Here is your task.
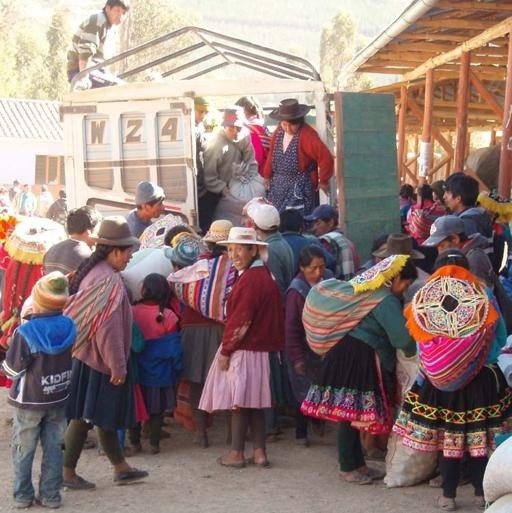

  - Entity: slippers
[245,455,270,468]
[216,454,246,468]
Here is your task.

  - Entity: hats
[165,198,340,264]
[88,216,142,250]
[32,269,71,313]
[370,234,424,263]
[425,214,467,246]
[221,106,248,128]
[267,99,311,123]
[134,180,165,205]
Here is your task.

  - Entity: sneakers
[34,492,62,509]
[113,467,149,485]
[62,475,97,491]
[59,418,324,453]
[14,493,34,508]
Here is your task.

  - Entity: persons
[67,0,129,90]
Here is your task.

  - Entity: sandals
[429,464,484,512]
[360,444,386,461]
[373,439,387,450]
[340,471,372,485]
[357,464,387,479]
